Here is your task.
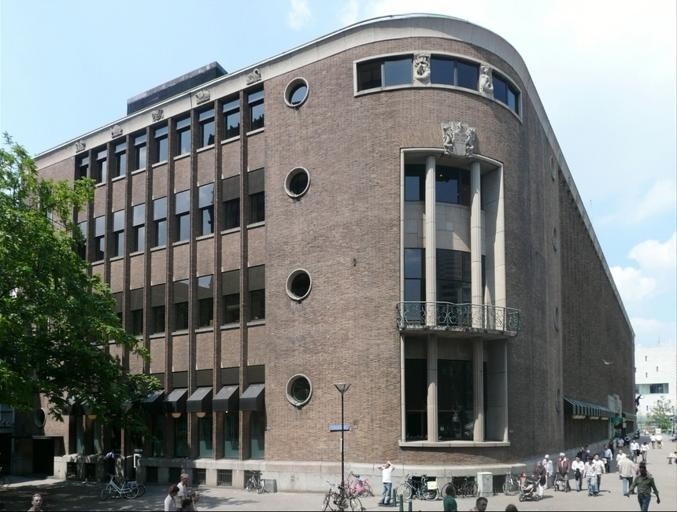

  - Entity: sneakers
[576,489,599,496]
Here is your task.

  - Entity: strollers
[552,470,572,493]
[516,474,545,502]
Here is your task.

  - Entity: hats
[545,454,549,459]
[560,452,565,457]
[181,474,189,479]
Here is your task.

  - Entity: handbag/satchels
[182,495,193,509]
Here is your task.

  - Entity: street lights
[331,382,352,498]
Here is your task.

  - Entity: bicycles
[440,475,479,500]
[395,473,440,503]
[498,470,532,497]
[321,470,371,512]
[97,471,146,500]
[244,468,266,494]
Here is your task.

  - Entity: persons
[175,473,200,512]
[27,493,44,511]
[443,486,457,511]
[469,497,488,512]
[537,429,676,511]
[164,484,179,512]
[505,504,517,512]
[378,459,395,504]
